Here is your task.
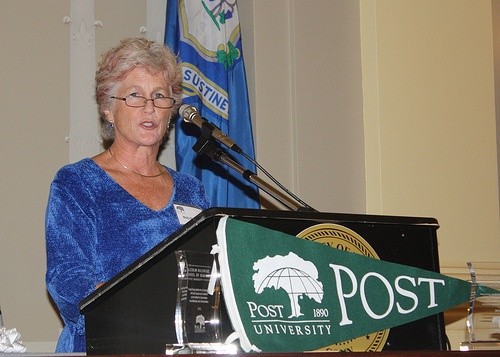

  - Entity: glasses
[111,94,177,108]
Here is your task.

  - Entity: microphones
[179,104,241,153]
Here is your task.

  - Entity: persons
[44,38,216,353]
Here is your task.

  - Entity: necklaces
[108,147,165,177]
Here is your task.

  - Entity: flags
[162,0,262,210]
